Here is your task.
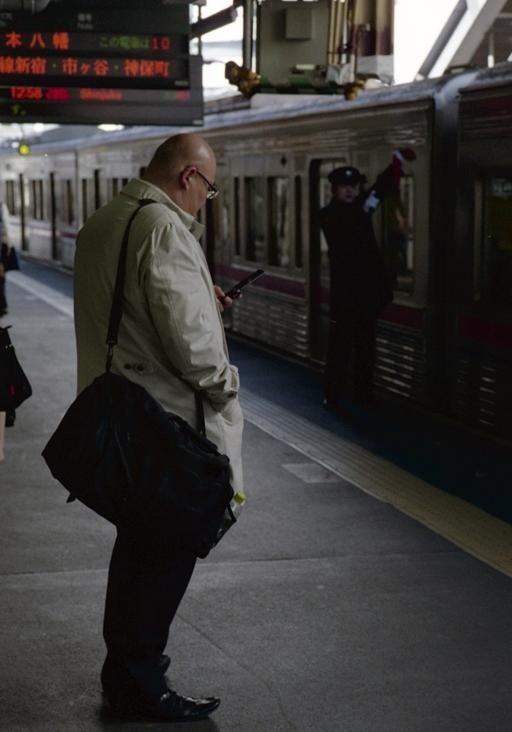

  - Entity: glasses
[196,171,220,199]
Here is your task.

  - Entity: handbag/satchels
[40,373,236,559]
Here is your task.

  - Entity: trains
[0,63,512,451]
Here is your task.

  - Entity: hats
[328,166,362,185]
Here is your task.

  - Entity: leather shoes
[100,687,223,719]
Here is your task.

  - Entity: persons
[1,200,33,464]
[67,128,253,723]
[310,141,421,426]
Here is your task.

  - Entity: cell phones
[217,269,265,304]
